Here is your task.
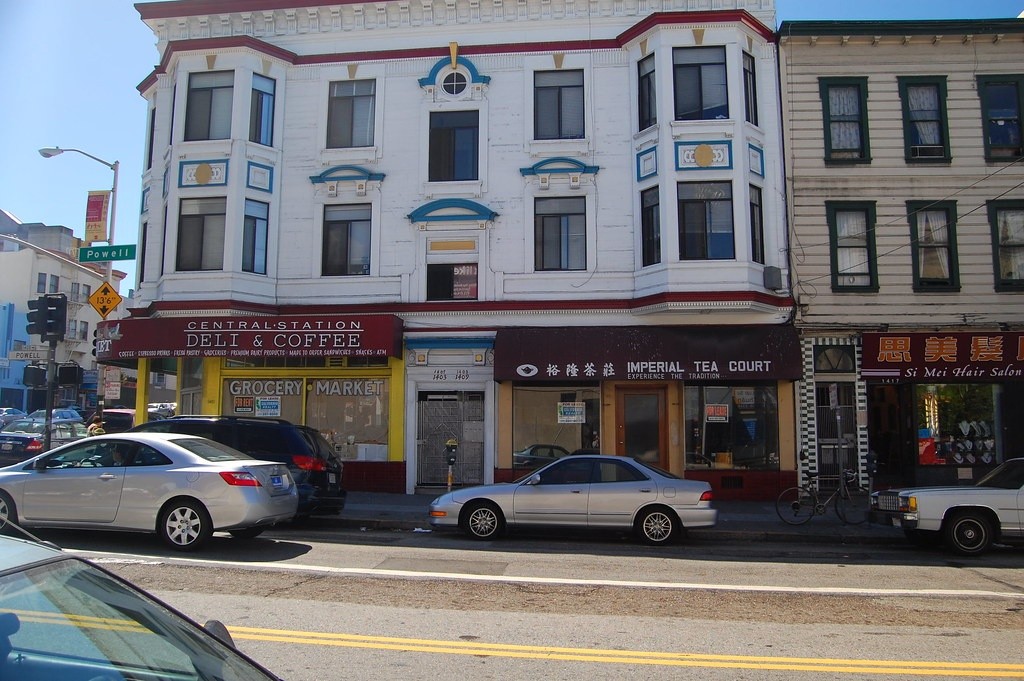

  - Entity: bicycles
[775,468,872,524]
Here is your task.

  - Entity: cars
[0,418,6,429]
[0,407,28,424]
[0,417,90,458]
[869,457,1024,557]
[0,432,299,552]
[148,402,177,418]
[0,534,283,681]
[513,442,570,470]
[429,454,718,546]
[920,442,969,465]
[85,409,167,437]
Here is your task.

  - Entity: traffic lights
[25,294,66,342]
[91,329,98,356]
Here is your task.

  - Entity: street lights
[40,148,118,422]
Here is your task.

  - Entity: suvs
[124,413,346,529]
[26,409,84,424]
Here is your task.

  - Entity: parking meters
[865,450,878,512]
[447,439,457,491]
